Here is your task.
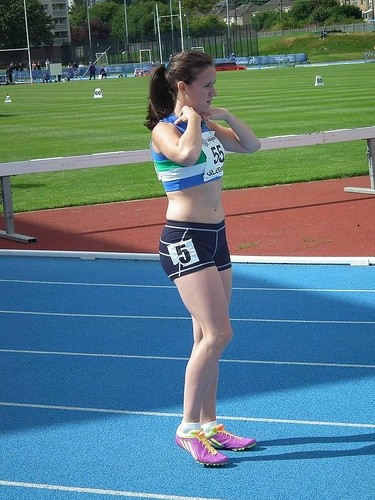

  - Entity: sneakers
[175,424,256,465]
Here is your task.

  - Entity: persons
[100,67,107,79]
[6,61,24,85]
[88,62,96,80]
[68,62,85,68]
[27,61,43,70]
[144,50,261,466]
[42,71,49,83]
[45,58,55,69]
